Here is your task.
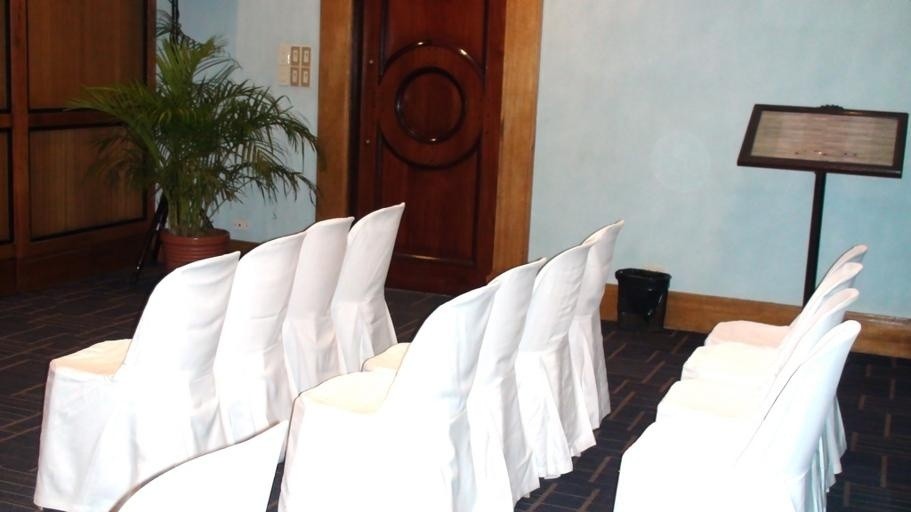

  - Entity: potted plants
[62,0,326,277]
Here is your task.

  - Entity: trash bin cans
[615,268,672,331]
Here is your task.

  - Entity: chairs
[34,202,414,512]
[109,218,636,512]
[618,245,867,512]
